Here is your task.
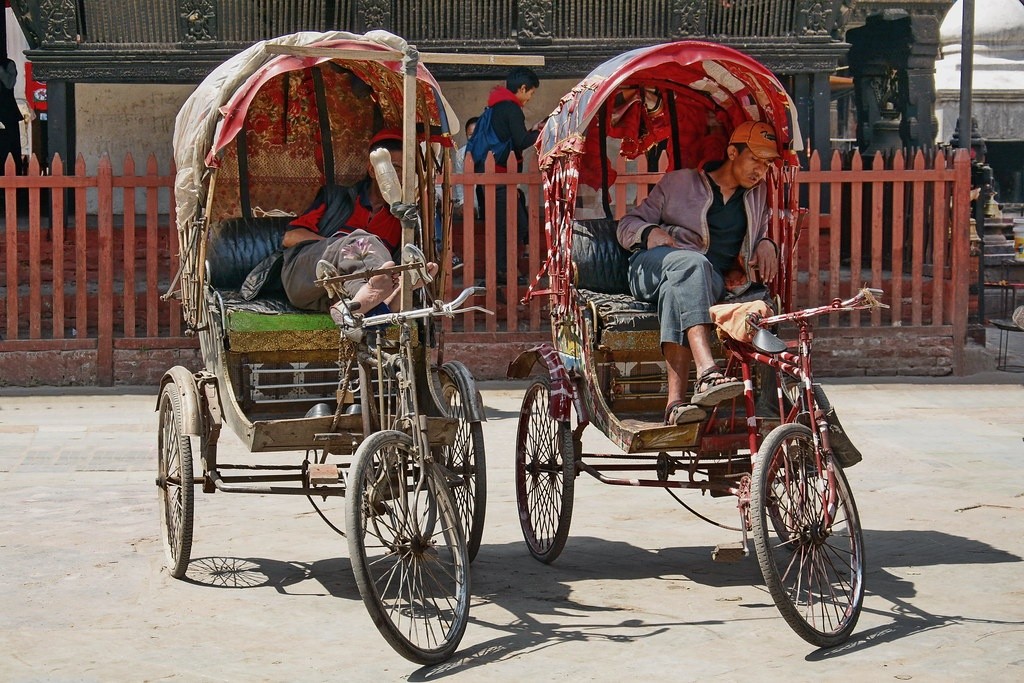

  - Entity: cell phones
[540,116,549,124]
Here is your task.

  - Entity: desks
[984,282,1024,321]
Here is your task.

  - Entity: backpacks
[465,106,512,173]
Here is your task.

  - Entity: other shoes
[498,269,527,286]
[436,256,464,271]
[304,404,331,418]
[345,403,361,414]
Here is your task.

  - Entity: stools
[990,321,1024,373]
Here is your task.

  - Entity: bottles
[369,147,402,207]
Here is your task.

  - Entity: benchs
[558,218,783,350]
[207,216,419,353]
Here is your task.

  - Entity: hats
[729,121,781,159]
[368,127,403,151]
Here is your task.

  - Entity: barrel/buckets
[1012,218,1024,262]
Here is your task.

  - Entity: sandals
[690,366,745,406]
[663,400,707,425]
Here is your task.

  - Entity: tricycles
[154,28,494,666]
[507,42,866,648]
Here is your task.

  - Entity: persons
[280,128,439,314]
[434,66,545,286]
[617,120,781,426]
